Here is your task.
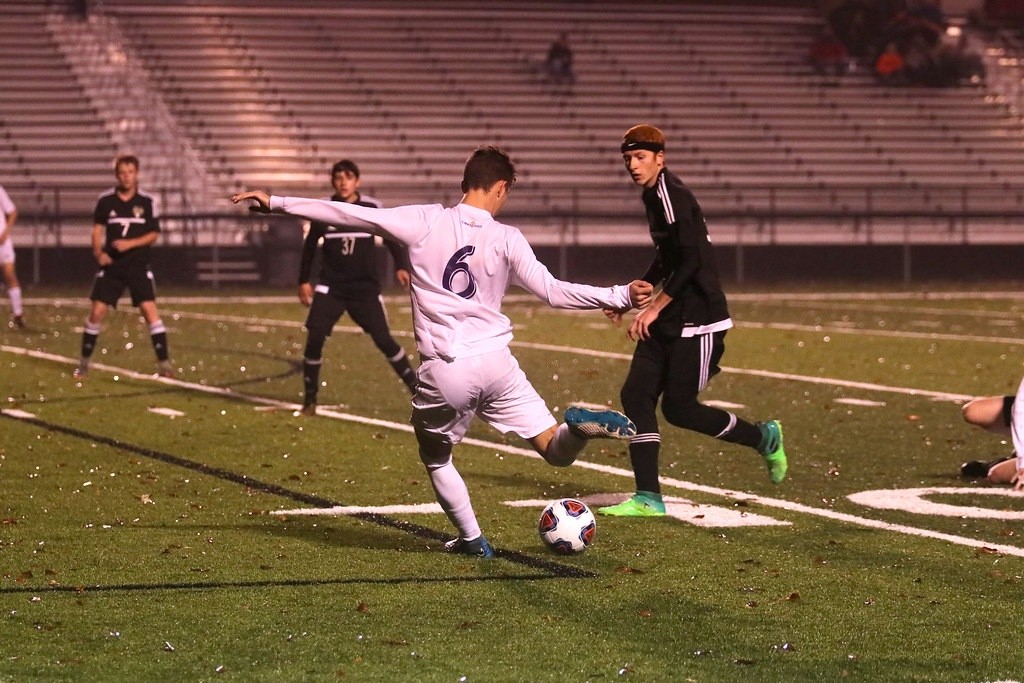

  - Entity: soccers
[539,498,597,552]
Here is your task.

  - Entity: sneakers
[597,490,666,517]
[564,401,637,440]
[753,420,789,484]
[444,533,493,558]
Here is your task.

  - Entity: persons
[544,30,577,96]
[0,183,29,330]
[72,154,175,381]
[597,124,790,518]
[298,158,421,417]
[958,375,1024,493]
[229,143,652,556]
[812,0,987,90]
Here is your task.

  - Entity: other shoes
[302,404,315,416]
[12,316,30,334]
[159,362,173,379]
[74,365,88,380]
[961,456,1009,478]
